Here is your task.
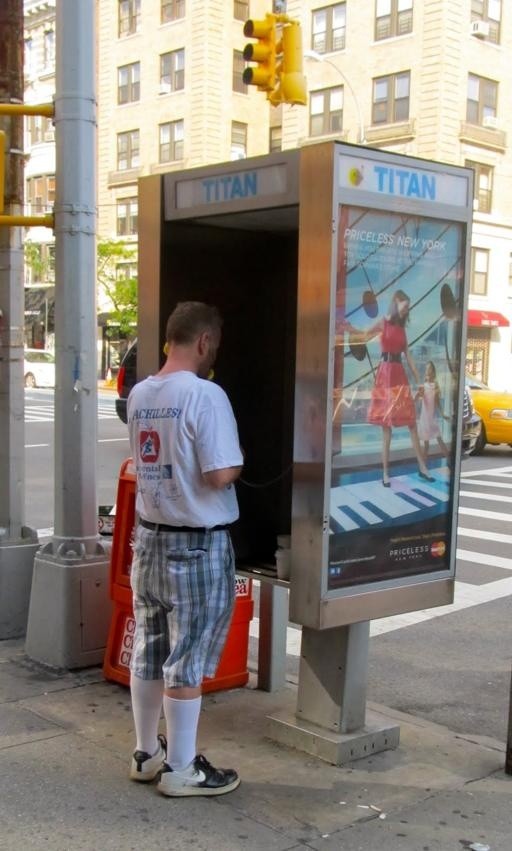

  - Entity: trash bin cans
[102,455,255,695]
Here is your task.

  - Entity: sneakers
[130,734,167,780]
[154,755,241,796]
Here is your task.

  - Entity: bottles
[274,535,292,580]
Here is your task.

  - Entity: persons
[125,302,252,796]
[417,351,453,463]
[342,288,435,486]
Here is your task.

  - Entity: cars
[24,347,56,389]
[463,373,512,457]
[114,337,136,424]
[462,387,483,462]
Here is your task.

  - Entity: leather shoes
[419,471,435,482]
[383,480,390,487]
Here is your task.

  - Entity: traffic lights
[239,12,276,92]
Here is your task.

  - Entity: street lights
[306,49,366,144]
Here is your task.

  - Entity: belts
[139,517,227,532]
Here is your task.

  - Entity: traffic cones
[102,367,113,387]
